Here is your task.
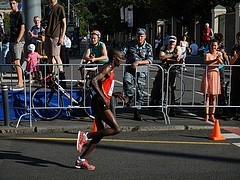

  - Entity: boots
[134,109,142,121]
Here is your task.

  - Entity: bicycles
[31,66,97,121]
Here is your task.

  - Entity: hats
[136,28,146,35]
[27,44,36,50]
[168,35,177,42]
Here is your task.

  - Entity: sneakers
[75,156,96,170]
[76,130,92,153]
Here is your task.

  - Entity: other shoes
[8,85,24,91]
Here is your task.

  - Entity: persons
[75,30,107,87]
[74,48,123,170]
[155,23,240,122]
[121,28,153,121]
[0,0,71,91]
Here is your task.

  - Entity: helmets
[91,30,101,37]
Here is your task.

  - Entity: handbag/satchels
[63,35,71,48]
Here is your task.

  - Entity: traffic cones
[91,118,98,132]
[207,119,226,141]
